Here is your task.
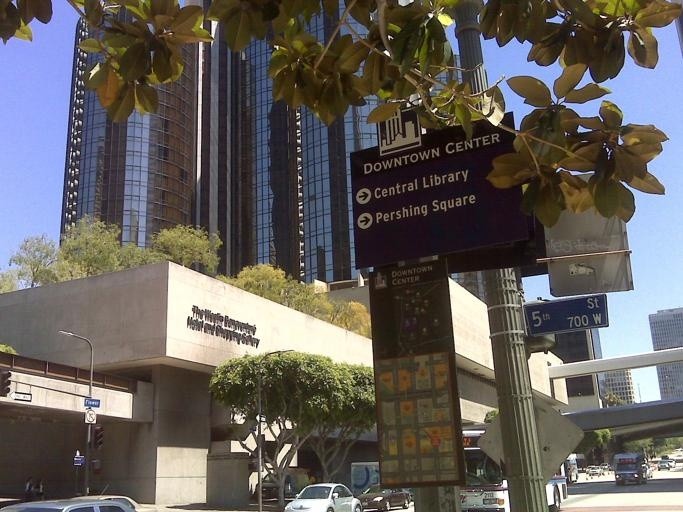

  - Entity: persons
[37,476,48,501]
[24,476,33,502]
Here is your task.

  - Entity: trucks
[615,450,647,485]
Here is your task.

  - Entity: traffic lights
[93,423,104,448]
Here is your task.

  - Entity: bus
[460,423,567,512]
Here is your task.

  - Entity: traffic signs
[351,105,536,271]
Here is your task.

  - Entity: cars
[0,494,185,512]
[283,482,362,511]
[586,465,602,477]
[358,484,411,510]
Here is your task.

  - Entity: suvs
[658,460,670,470]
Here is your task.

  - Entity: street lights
[59,330,94,493]
[259,349,296,511]
